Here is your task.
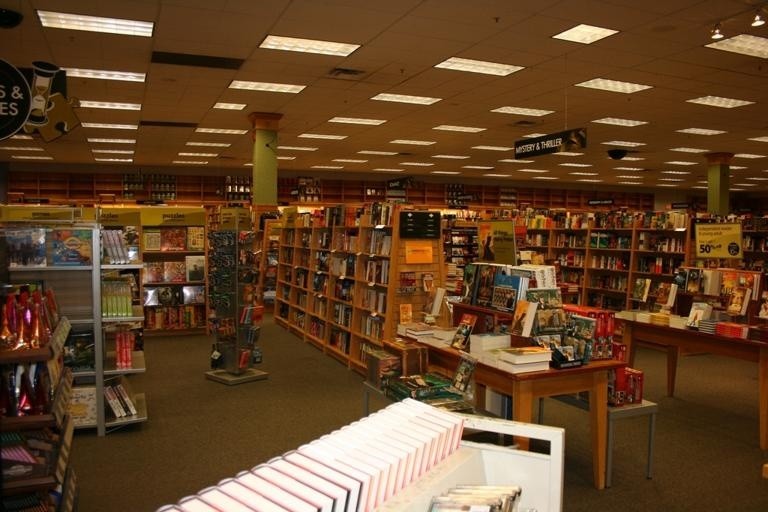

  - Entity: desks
[538,392,658,487]
[362,381,500,448]
[623,318,768,451]
[397,327,627,490]
[449,301,515,334]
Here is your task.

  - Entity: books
[164,396,523,512]
[4,221,137,427]
[206,177,767,412]
[143,226,206,329]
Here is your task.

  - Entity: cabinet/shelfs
[0,315,77,512]
[273,210,448,379]
[0,228,100,436]
[158,397,565,512]
[7,171,252,206]
[249,219,282,312]
[514,227,717,356]
[97,229,147,436]
[143,225,209,336]
[204,218,268,385]
[441,227,525,271]
[718,229,768,342]
[277,176,655,212]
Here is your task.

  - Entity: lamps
[711,23,724,39]
[751,7,765,27]
